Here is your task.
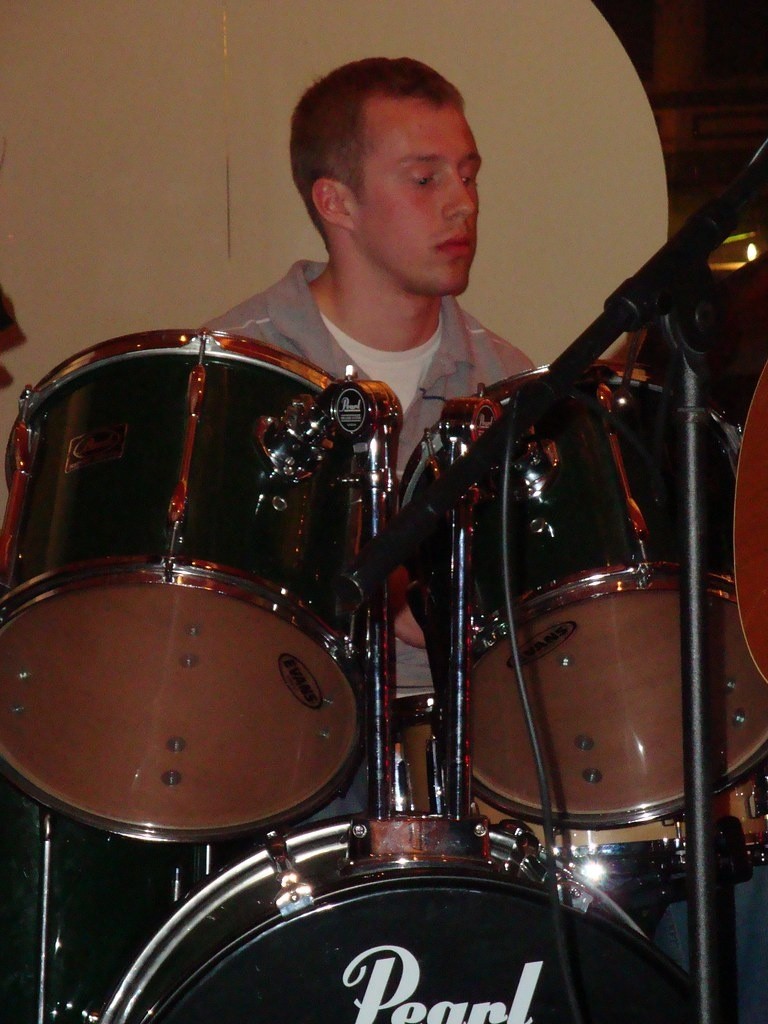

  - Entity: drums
[420,689,768,887]
[0,330,390,845]
[97,810,698,1023]
[392,360,768,827]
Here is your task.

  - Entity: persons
[207,58,549,698]
